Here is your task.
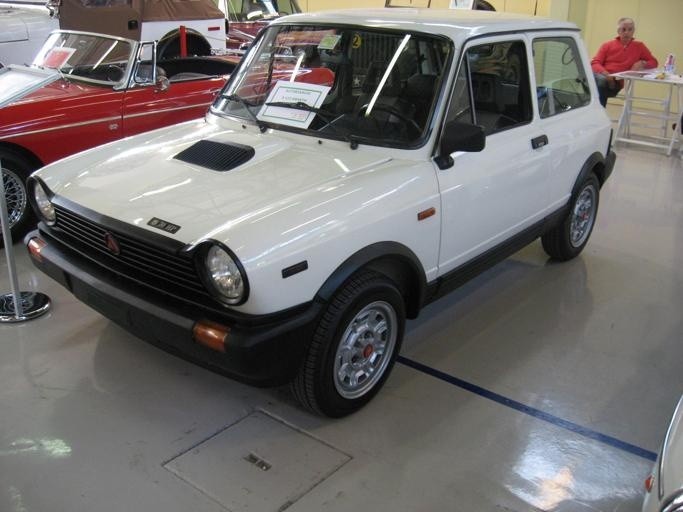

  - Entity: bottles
[664,54,674,73]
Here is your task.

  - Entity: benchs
[607,80,672,143]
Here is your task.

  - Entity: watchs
[641,58,648,67]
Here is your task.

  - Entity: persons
[590,16,658,108]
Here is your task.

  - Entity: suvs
[23,7,618,421]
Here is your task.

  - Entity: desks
[608,70,683,157]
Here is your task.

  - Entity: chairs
[137,65,209,82]
[334,62,504,139]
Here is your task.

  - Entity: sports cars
[0,27,337,251]
